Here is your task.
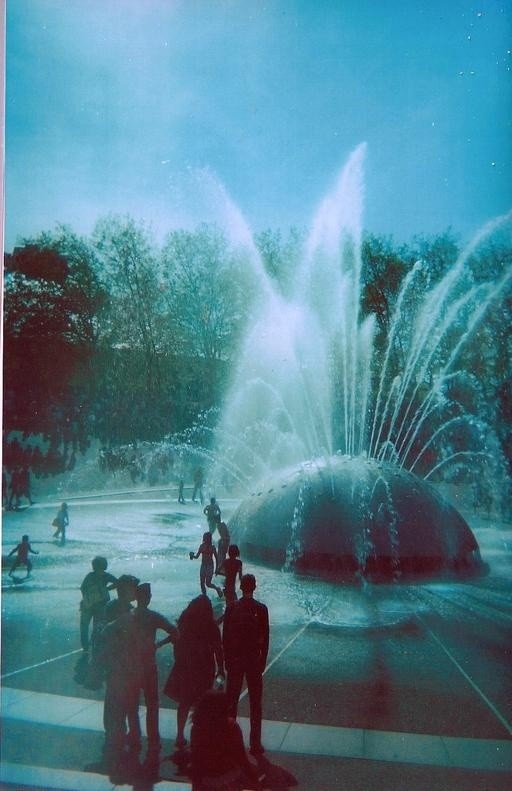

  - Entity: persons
[95,574,140,763]
[218,571,271,756]
[3,393,200,514]
[185,689,256,790]
[187,532,223,599]
[77,556,118,652]
[191,467,206,506]
[215,544,243,605]
[202,497,222,537]
[92,582,181,785]
[53,502,70,545]
[7,534,40,579]
[160,593,227,747]
[216,522,231,570]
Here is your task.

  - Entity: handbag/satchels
[82,584,110,613]
